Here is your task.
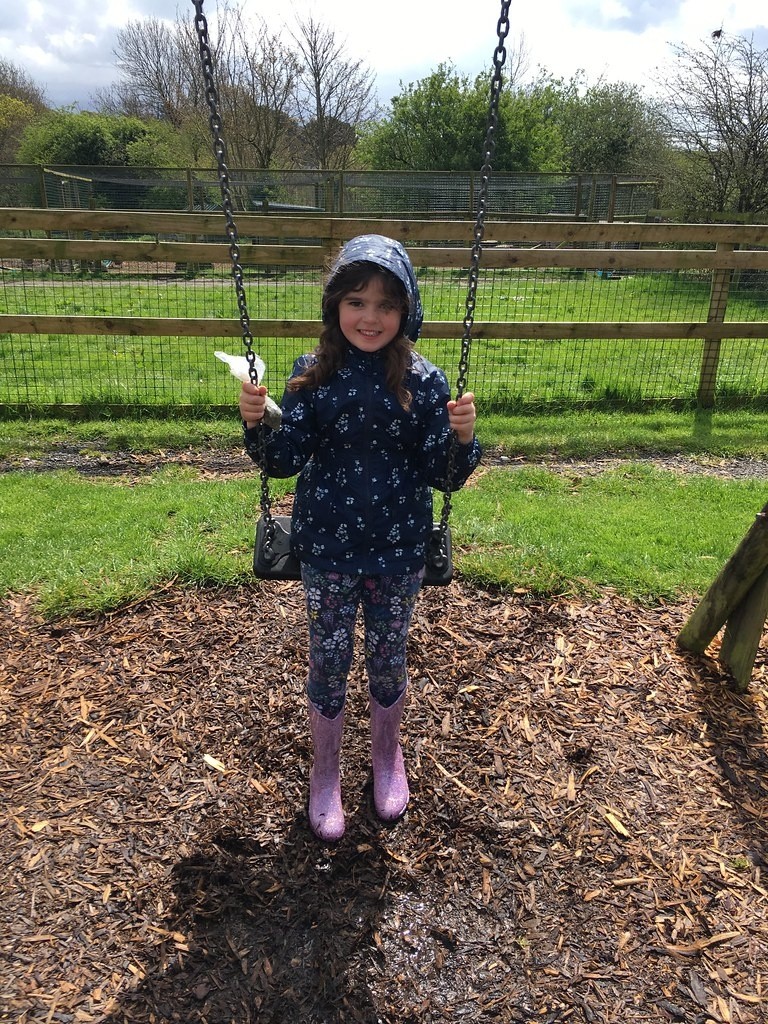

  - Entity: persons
[239,232,483,842]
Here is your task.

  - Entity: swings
[191,1,515,585]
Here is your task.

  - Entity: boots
[307,693,345,840]
[368,672,409,818]
